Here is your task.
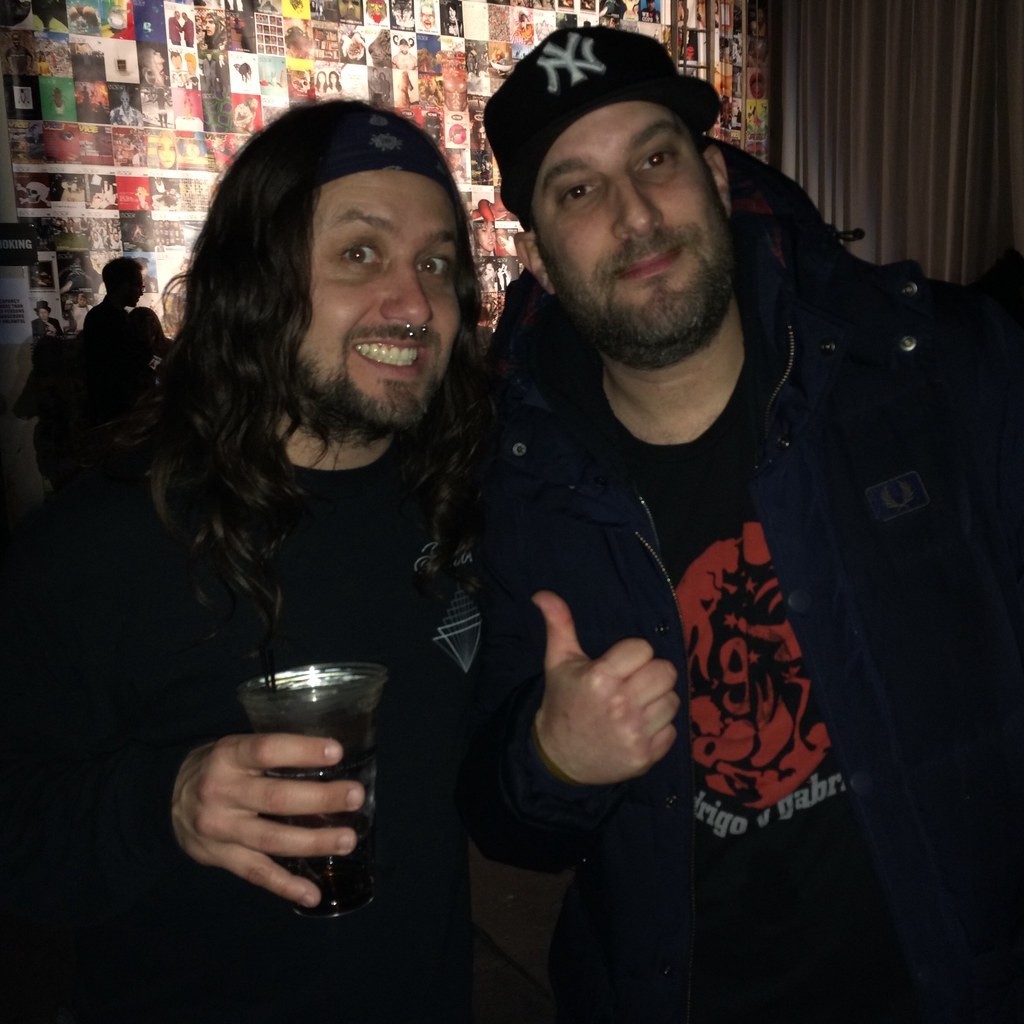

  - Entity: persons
[470,25,1024,1024]
[0,100,495,1023]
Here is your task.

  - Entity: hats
[483,25,719,233]
[34,300,51,312]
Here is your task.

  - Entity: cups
[242,664,383,918]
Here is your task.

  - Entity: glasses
[134,285,145,294]
